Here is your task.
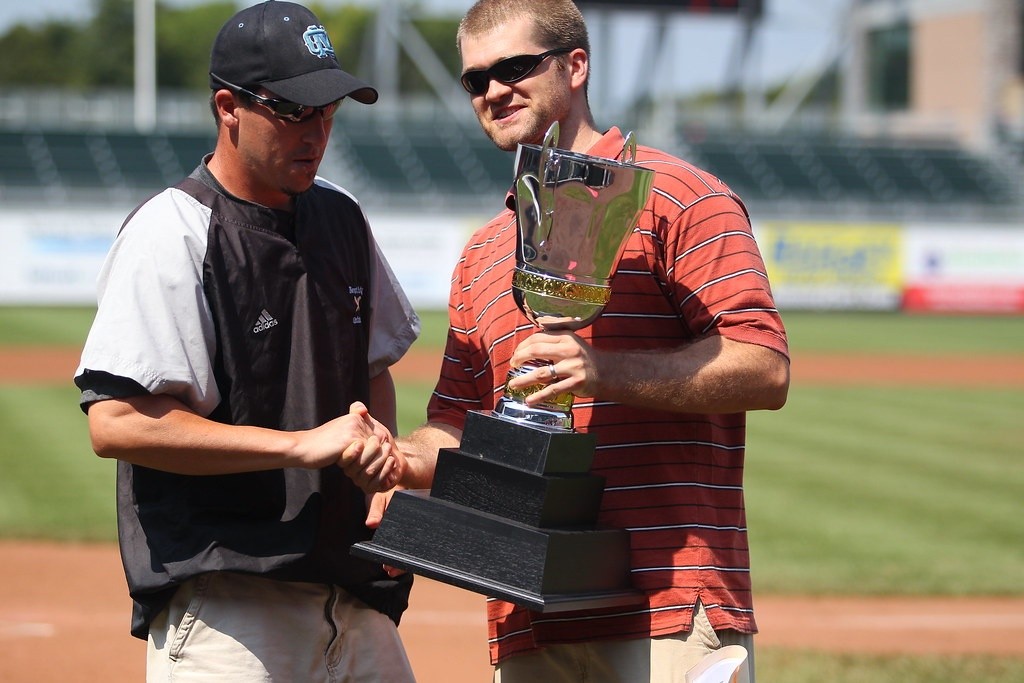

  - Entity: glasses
[211,72,341,122]
[461,45,581,95]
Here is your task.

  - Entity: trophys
[350,114,656,614]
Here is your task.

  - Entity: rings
[549,364,558,380]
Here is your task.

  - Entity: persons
[75,0,420,683]
[337,1,790,683]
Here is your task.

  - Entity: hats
[210,1,379,105]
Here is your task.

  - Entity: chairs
[2,128,1022,222]
